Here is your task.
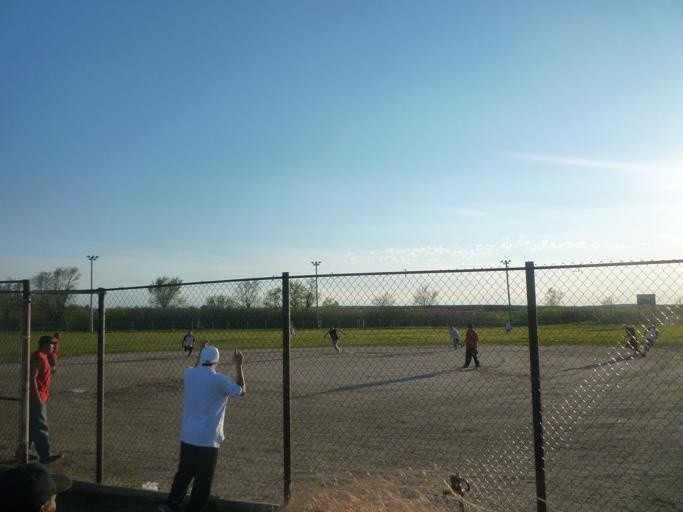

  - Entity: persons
[13,335,64,464]
[460,322,480,369]
[158,338,245,511]
[504,318,511,334]
[619,323,646,358]
[643,323,659,351]
[50,332,59,374]
[322,324,346,354]
[181,329,195,358]
[446,323,462,349]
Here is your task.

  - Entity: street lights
[86,255,99,333]
[500,260,512,329]
[310,261,321,329]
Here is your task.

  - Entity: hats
[198,344,221,367]
[36,335,58,345]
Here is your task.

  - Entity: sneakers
[16,451,64,463]
